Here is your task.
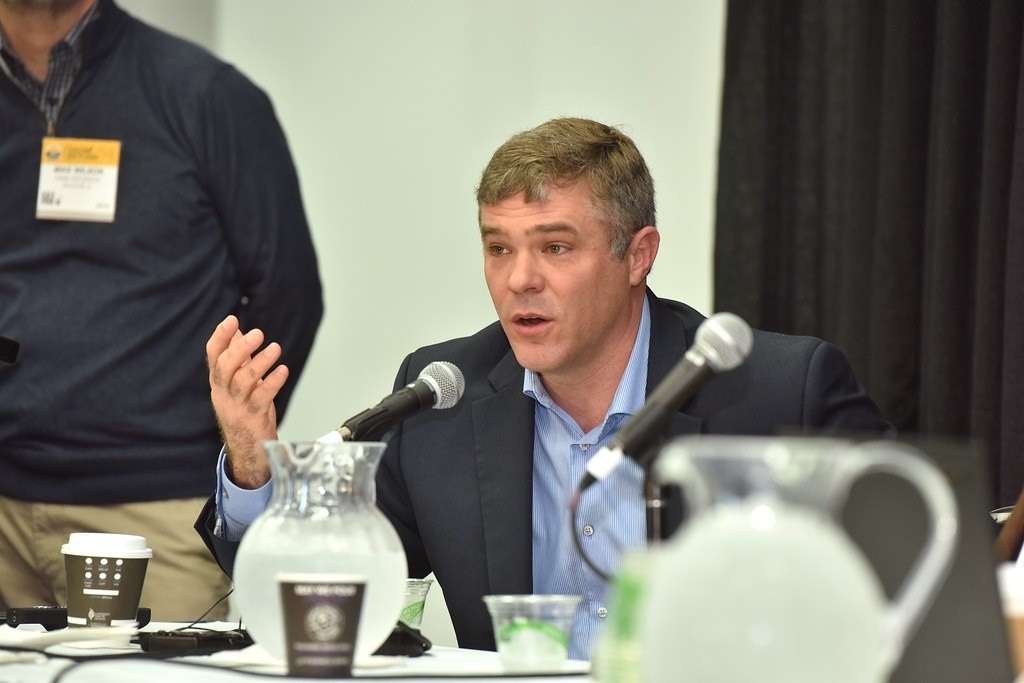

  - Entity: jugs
[635,434,962,683]
[231,441,408,661]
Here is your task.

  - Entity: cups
[398,579,433,629]
[60,531,153,627]
[482,593,584,667]
[273,570,369,676]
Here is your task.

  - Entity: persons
[0,0,325,624]
[193,118,894,662]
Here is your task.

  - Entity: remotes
[139,629,253,656]
[6,606,151,631]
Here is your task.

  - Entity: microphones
[335,361,465,443]
[574,311,755,493]
